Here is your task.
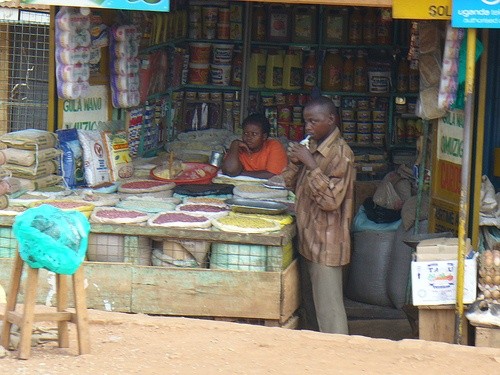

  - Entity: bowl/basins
[150,162,218,186]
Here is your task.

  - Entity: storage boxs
[411,238,478,306]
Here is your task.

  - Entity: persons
[222,114,288,179]
[282,96,354,335]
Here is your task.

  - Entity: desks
[418,306,469,345]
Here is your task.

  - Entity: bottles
[248,0,421,96]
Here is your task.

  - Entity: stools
[1,203,90,359]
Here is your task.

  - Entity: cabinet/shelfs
[1,0,431,328]
